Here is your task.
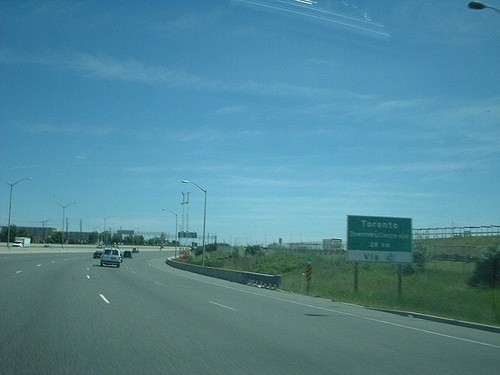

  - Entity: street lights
[96,214,114,244]
[0,177,32,247]
[181,180,207,266]
[54,201,77,248]
[161,207,178,259]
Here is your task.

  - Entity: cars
[123,250,132,259]
[93,251,103,259]
[132,248,139,253]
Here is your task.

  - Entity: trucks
[100,248,123,268]
[13,236,31,248]
